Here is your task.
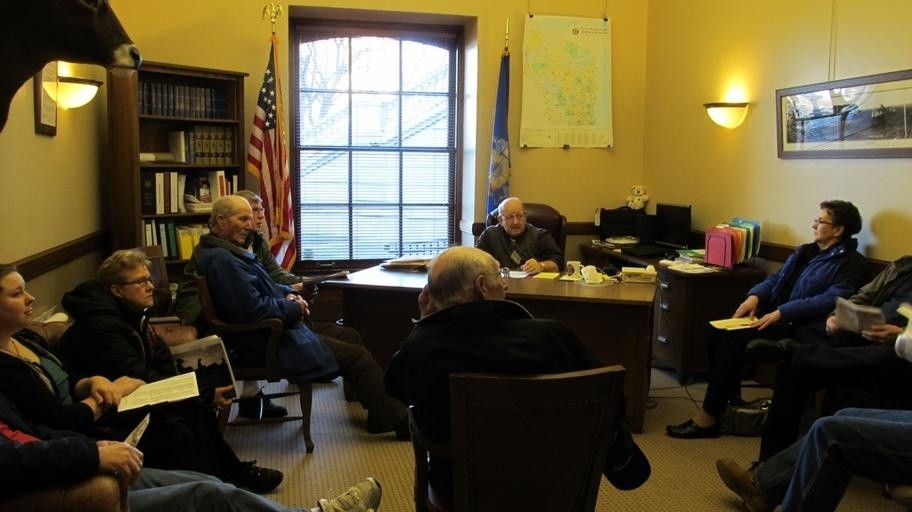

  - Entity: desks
[322,251,664,435]
[578,227,765,386]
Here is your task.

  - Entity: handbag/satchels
[721,396,773,436]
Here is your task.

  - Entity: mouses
[665,252,676,261]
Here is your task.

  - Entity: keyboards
[626,245,666,258]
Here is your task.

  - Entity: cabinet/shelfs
[103,59,250,301]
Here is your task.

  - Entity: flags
[485,17,514,226]
[245,27,303,273]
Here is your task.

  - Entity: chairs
[136,244,170,301]
[484,198,570,271]
[190,265,325,454]
[407,358,628,512]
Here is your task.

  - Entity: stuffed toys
[623,184,651,210]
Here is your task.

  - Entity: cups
[500,267,511,280]
[566,260,584,278]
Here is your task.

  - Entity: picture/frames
[775,67,912,160]
[30,59,62,139]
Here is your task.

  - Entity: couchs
[1,313,199,510]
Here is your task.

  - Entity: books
[662,218,763,276]
[133,68,240,264]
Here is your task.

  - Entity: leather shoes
[666,418,722,440]
[716,458,773,512]
[368,416,397,434]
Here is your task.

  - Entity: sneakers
[239,386,288,420]
[227,460,285,495]
[317,475,382,512]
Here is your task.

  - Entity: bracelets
[540,262,545,272]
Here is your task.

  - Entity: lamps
[702,99,751,130]
[41,75,103,111]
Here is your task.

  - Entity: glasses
[473,267,510,286]
[114,276,156,287]
[813,217,839,227]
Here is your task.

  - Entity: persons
[665,199,867,438]
[716,254,912,511]
[0,247,382,511]
[382,245,651,512]
[186,190,411,441]
[474,197,565,276]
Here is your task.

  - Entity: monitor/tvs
[655,200,692,250]
